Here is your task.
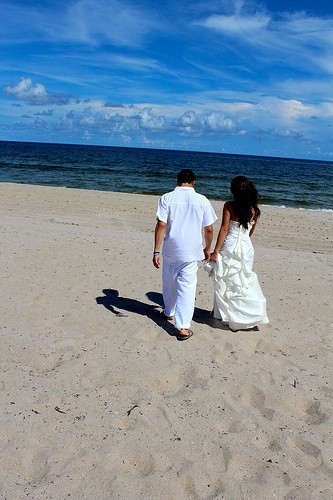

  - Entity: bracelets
[153,252,159,253]
[154,253,159,256]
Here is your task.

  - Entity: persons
[152,169,218,341]
[210,175,269,332]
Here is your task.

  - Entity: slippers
[178,329,193,340]
[163,308,173,320]
